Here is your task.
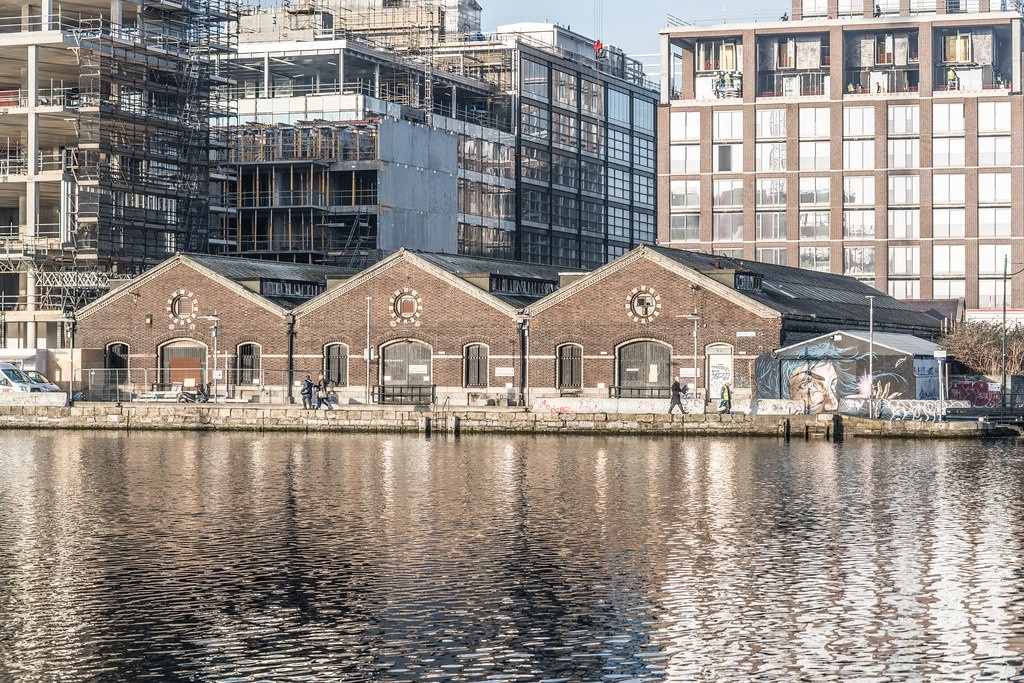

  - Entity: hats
[305,375,310,380]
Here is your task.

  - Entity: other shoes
[682,412,690,414]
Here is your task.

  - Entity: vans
[0,363,62,393]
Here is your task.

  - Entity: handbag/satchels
[301,387,311,395]
[681,385,689,395]
[716,399,727,412]
[318,387,327,397]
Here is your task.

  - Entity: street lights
[284,311,297,404]
[366,297,371,404]
[865,295,874,419]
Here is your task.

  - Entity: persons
[721,380,734,414]
[668,376,689,414]
[301,375,314,410]
[313,374,333,410]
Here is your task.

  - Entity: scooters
[177,384,209,403]
